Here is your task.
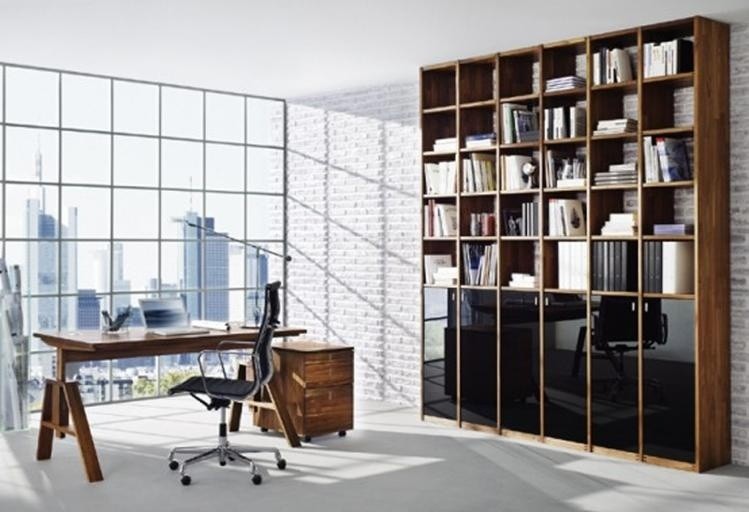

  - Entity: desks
[33,321,308,483]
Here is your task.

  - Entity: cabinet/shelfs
[420,16,730,473]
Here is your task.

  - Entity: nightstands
[254,341,354,443]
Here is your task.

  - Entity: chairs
[167,282,287,485]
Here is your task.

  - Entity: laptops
[141,298,208,336]
[549,292,587,307]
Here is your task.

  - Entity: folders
[592,240,627,292]
[642,241,660,295]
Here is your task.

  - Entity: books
[642,137,690,182]
[644,40,693,78]
[544,147,586,189]
[461,154,496,192]
[461,242,496,287]
[425,162,457,194]
[654,223,692,236]
[544,52,587,92]
[466,133,495,147]
[502,200,538,236]
[508,273,540,288]
[592,242,636,293]
[600,212,637,237]
[594,164,636,184]
[424,253,457,285]
[431,137,458,151]
[503,103,538,145]
[544,104,585,141]
[592,119,637,135]
[593,43,638,85]
[557,241,589,290]
[501,155,539,190]
[643,242,694,295]
[422,198,456,236]
[547,198,585,236]
